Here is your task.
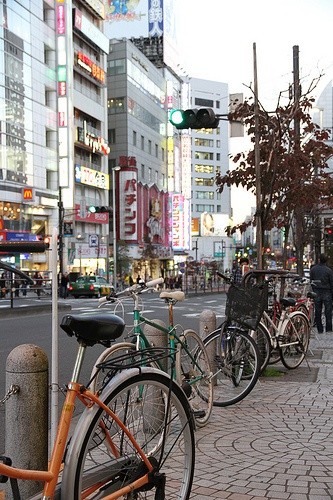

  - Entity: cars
[67,275,115,298]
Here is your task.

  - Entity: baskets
[225,285,267,330]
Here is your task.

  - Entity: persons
[310,254,333,333]
[236,258,254,284]
[0,271,70,300]
[115,273,182,290]
[203,213,214,235]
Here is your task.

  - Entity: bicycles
[189,269,324,406]
[89,277,223,463]
[0,313,197,500]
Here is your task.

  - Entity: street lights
[192,241,199,264]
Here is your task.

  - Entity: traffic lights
[246,246,250,250]
[87,206,108,213]
[170,107,219,130]
[323,227,333,235]
